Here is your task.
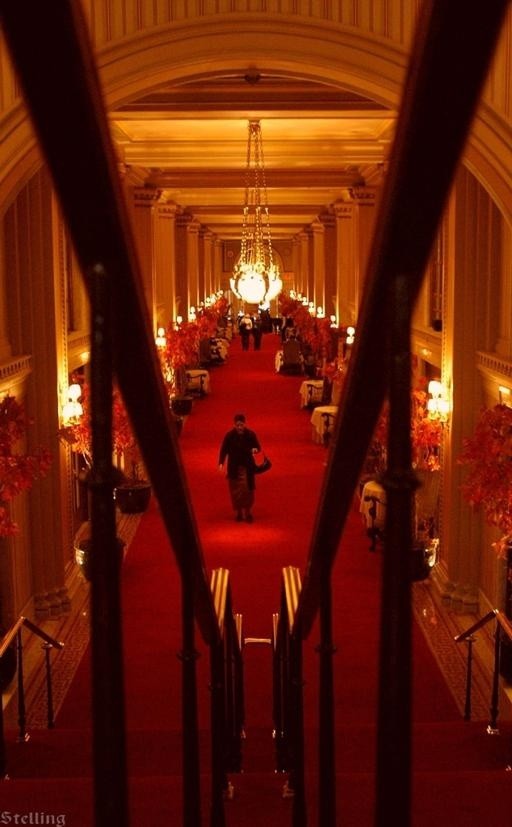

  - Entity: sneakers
[236,512,253,522]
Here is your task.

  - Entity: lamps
[228,118,285,305]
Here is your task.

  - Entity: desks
[361,478,390,534]
[298,375,342,445]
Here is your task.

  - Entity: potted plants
[155,319,201,416]
[55,357,153,514]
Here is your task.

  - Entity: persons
[251,313,262,351]
[219,415,260,523]
[239,313,253,351]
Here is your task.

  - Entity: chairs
[280,339,302,373]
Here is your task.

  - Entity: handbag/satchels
[252,454,272,474]
